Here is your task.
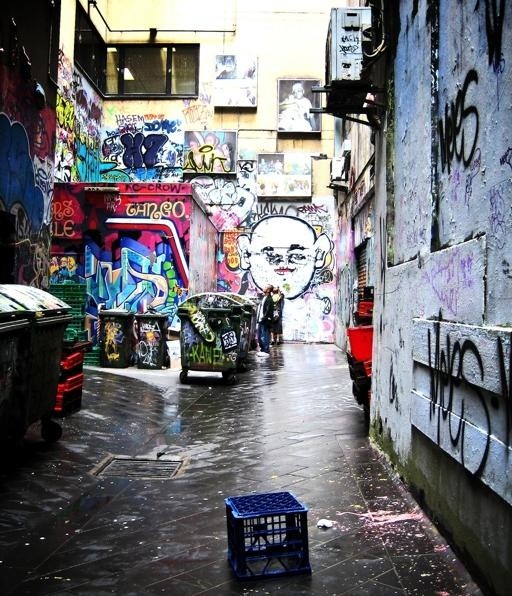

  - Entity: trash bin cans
[176,292,262,386]
[134,313,170,370]
[97,309,135,368]
[0,284,73,476]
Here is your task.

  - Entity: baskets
[224,491,311,583]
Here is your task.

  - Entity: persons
[189,132,234,173]
[271,287,284,345]
[216,55,236,79]
[279,83,317,133]
[259,158,283,174]
[256,284,274,353]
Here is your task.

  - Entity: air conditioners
[324,4,376,110]
